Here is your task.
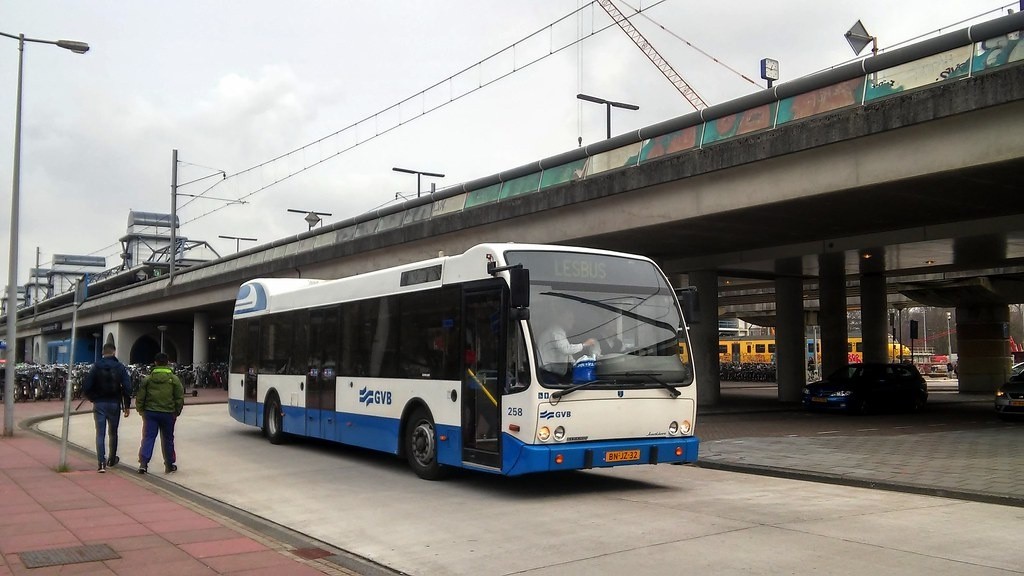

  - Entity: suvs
[800,363,928,417]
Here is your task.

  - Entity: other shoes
[97,461,106,473]
[165,464,177,474]
[107,456,119,467]
[137,463,147,474]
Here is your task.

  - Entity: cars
[1011,362,1024,375]
[994,368,1024,422]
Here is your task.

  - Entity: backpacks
[93,363,122,402]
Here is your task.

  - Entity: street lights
[3,32,90,438]
[219,235,257,252]
[576,93,639,140]
[286,208,332,232]
[392,167,445,197]
[946,312,953,378]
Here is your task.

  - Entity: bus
[224,241,700,482]
[677,334,914,367]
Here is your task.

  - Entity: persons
[807,356,816,381]
[136,353,184,475]
[946,361,959,378]
[539,307,629,384]
[82,342,131,472]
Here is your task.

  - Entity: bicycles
[719,361,778,383]
[0,361,229,404]
